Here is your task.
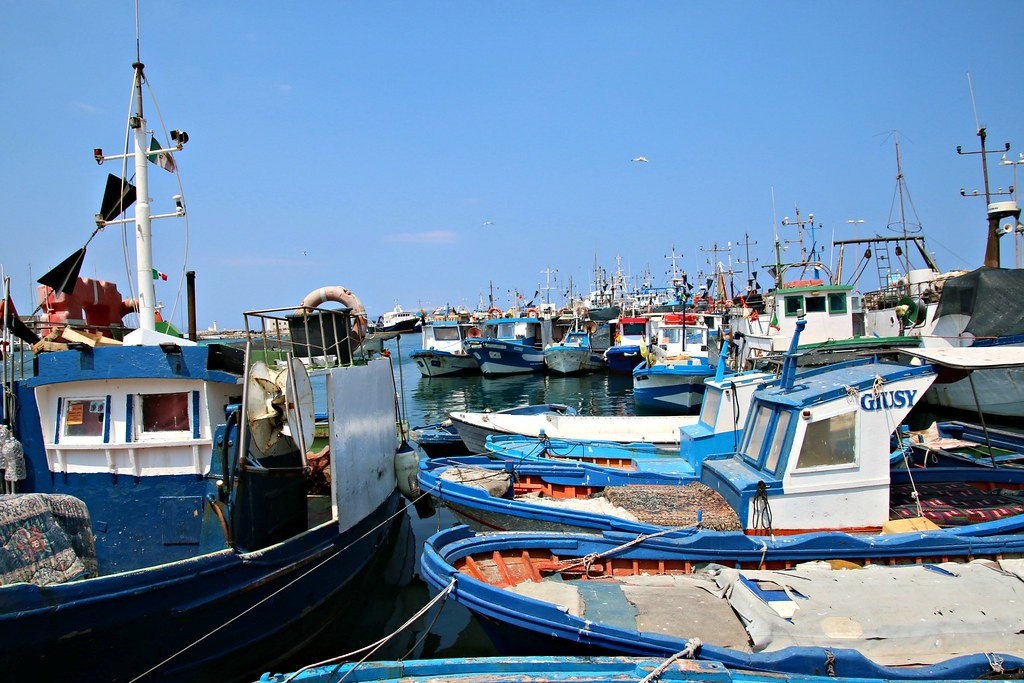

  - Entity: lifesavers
[693,295,715,314]
[527,308,539,319]
[487,307,502,320]
[559,307,569,315]
[295,285,368,351]
[715,301,729,315]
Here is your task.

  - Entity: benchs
[978,453,1024,466]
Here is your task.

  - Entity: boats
[0,0,1024,682]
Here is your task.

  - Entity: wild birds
[301,248,313,257]
[482,221,495,226]
[631,156,649,163]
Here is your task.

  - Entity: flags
[145,135,176,174]
[515,291,523,301]
[150,267,168,282]
[768,309,781,333]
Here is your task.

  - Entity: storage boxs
[123,327,197,348]
[31,325,123,355]
[882,516,943,536]
